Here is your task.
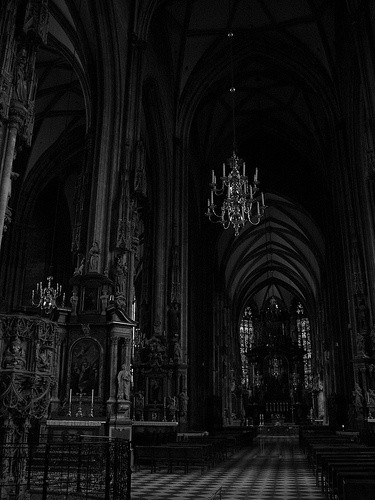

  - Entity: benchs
[116,424,374,499]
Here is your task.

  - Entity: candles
[30,276,68,318]
[204,147,269,234]
